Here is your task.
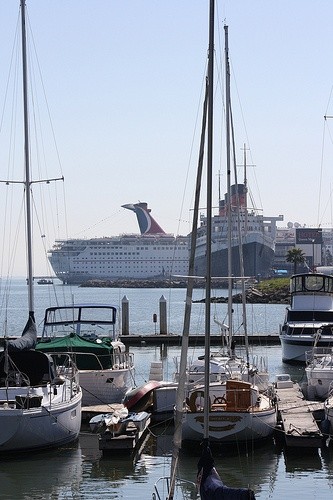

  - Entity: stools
[0,400,16,409]
[211,404,227,410]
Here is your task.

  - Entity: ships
[45,183,333,286]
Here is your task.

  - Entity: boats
[304,345,333,401]
[89,408,152,453]
[37,279,53,284]
[278,272,333,367]
[33,302,135,406]
[123,356,178,414]
[188,187,280,273]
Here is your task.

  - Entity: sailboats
[170,1,281,453]
[0,0,85,458]
